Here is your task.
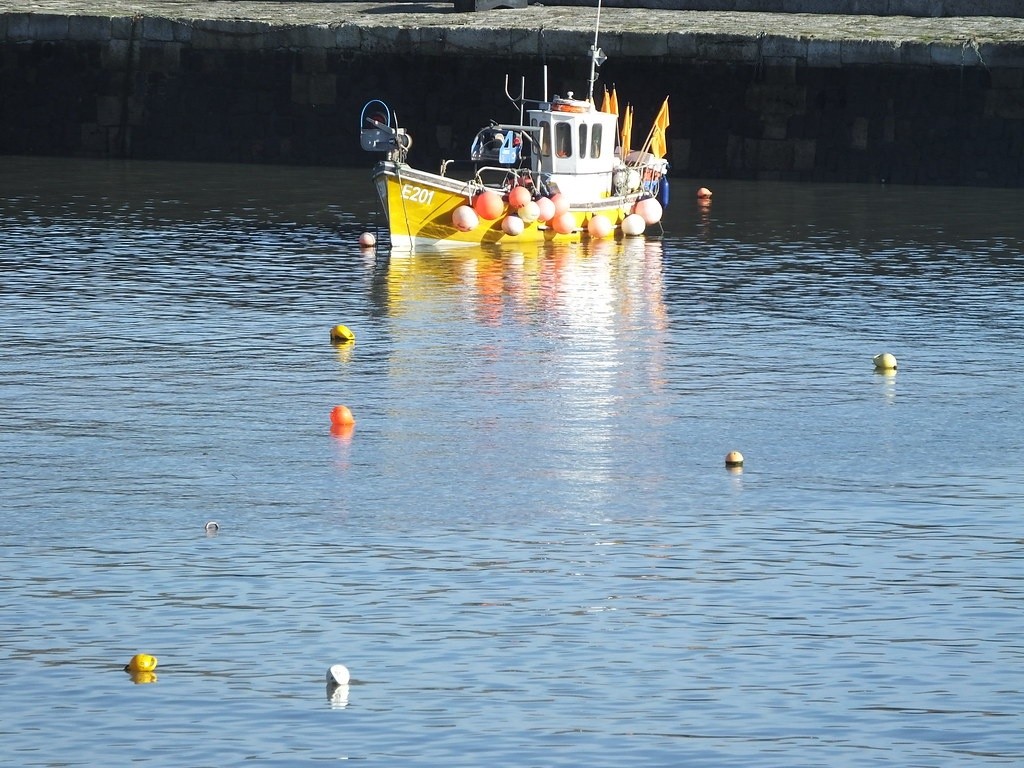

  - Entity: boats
[356,0,675,253]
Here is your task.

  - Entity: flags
[601,89,619,117]
[651,100,670,157]
[622,106,632,155]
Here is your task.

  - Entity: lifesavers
[551,103,589,113]
